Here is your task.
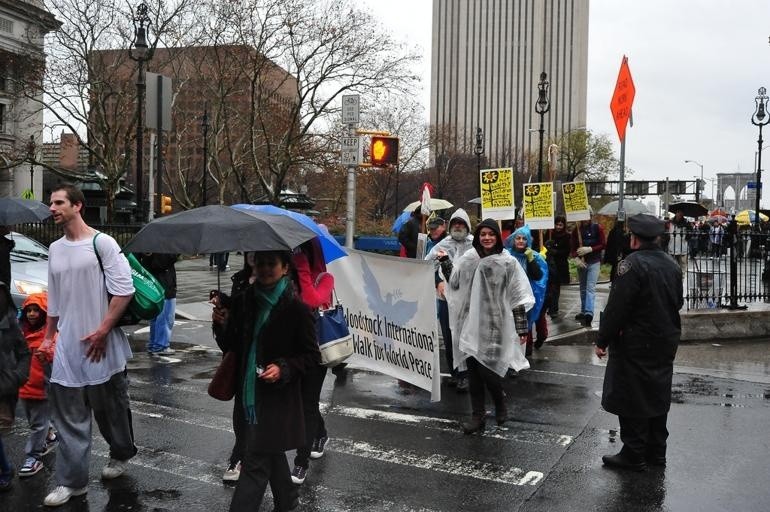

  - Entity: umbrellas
[226,197,349,268]
[0,194,52,250]
[120,201,318,325]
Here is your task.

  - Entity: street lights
[685,160,703,221]
[692,175,734,210]
[26,134,37,195]
[128,4,154,235]
[473,128,484,224]
[200,101,211,207]
[746,85,769,259]
[528,126,587,183]
[534,71,550,183]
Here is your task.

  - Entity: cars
[5,230,50,310]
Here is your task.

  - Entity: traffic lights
[369,136,400,166]
[161,194,172,214]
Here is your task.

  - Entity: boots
[494,396,507,423]
[464,414,486,435]
[602,443,667,471]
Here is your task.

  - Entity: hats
[427,217,444,229]
[628,213,665,242]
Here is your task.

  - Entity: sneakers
[44,485,88,506]
[290,465,307,484]
[448,376,470,391]
[102,457,130,478]
[534,335,547,349]
[0,464,17,489]
[18,455,44,476]
[576,314,592,326]
[148,347,176,355]
[551,311,558,318]
[31,433,60,456]
[310,436,329,458]
[223,457,242,481]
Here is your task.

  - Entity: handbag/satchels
[107,253,166,328]
[208,351,237,400]
[313,303,353,368]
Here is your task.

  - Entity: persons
[17,289,58,479]
[390,196,770,376]
[208,246,319,511]
[593,212,684,474]
[446,220,537,437]
[424,208,480,389]
[211,241,260,482]
[2,231,30,493]
[288,236,348,483]
[39,181,140,507]
[138,252,179,358]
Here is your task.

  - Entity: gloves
[524,247,534,263]
[573,257,587,269]
[576,246,592,256]
[540,246,548,259]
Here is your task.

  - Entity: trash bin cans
[686,268,726,310]
